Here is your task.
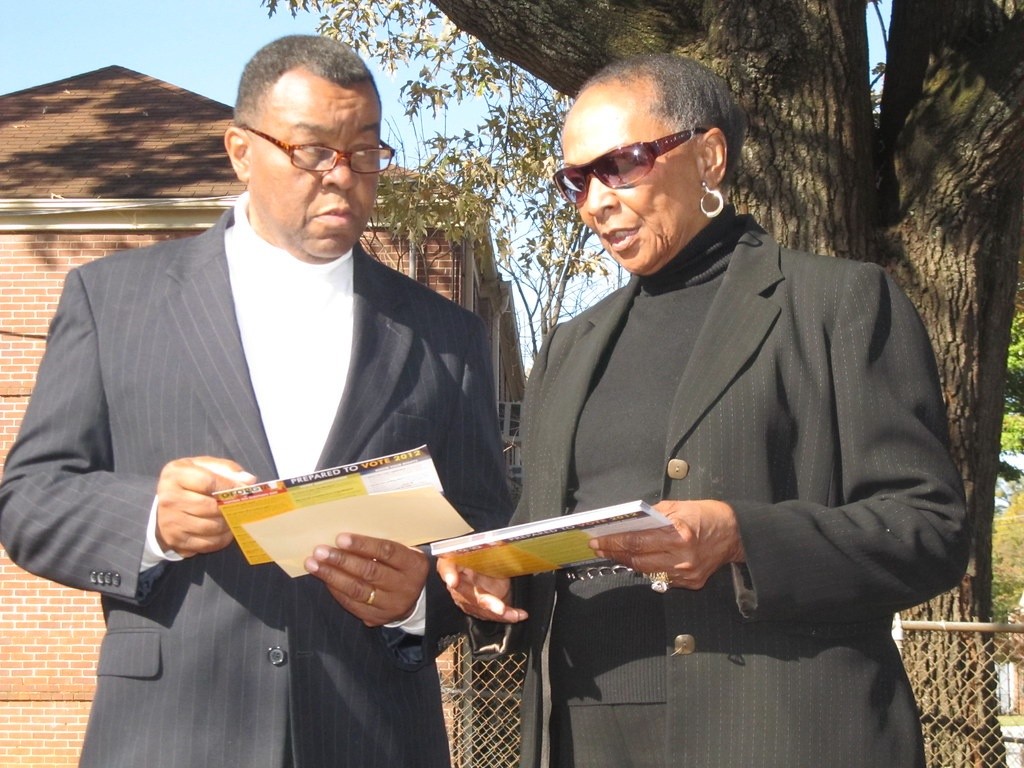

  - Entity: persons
[435,52,973,767]
[0,35,515,767]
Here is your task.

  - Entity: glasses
[553,127,708,205]
[237,124,396,174]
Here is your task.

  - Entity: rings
[367,586,376,605]
[650,572,671,593]
